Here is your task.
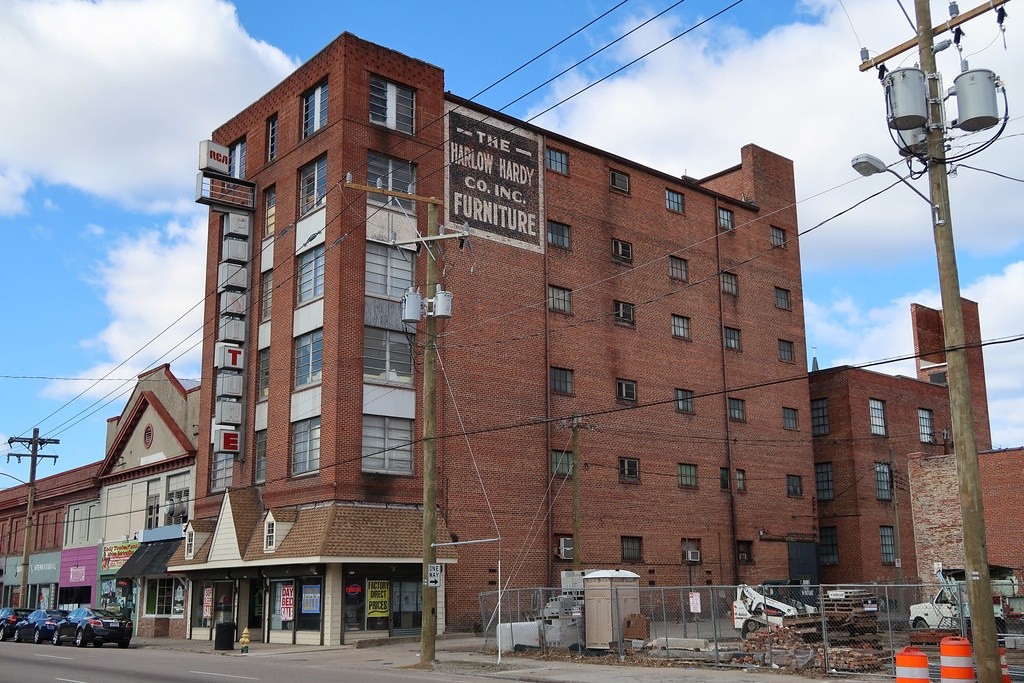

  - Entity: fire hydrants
[239,625,250,654]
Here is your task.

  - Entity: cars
[0,607,36,643]
[13,608,73,644]
[51,606,133,649]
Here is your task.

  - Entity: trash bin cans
[214,620,236,650]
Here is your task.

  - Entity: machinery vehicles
[908,564,1019,644]
[730,575,885,642]
